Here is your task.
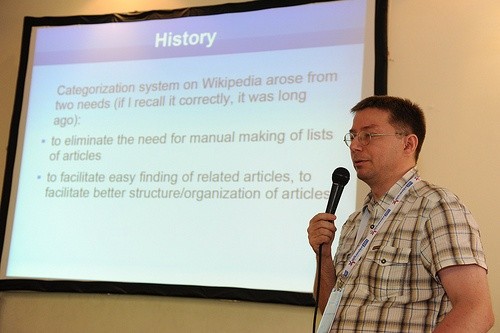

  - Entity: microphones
[324,167,350,223]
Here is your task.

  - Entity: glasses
[343,129,405,146]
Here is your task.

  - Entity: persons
[308,96,493,333]
